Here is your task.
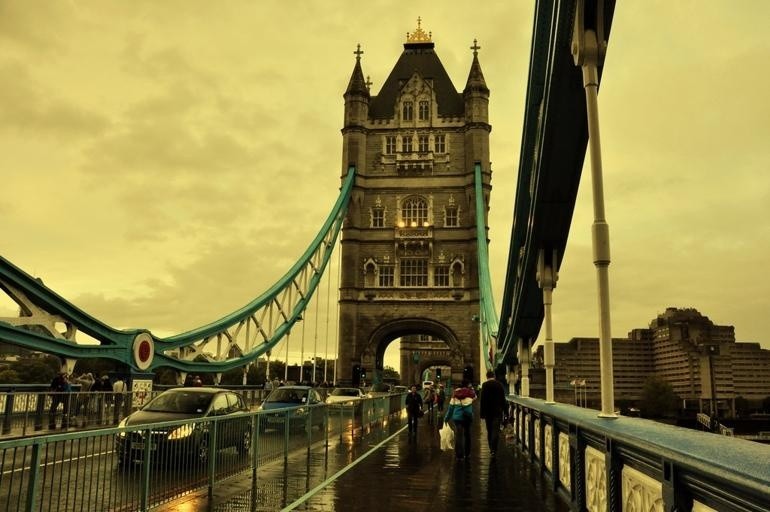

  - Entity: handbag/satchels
[461,410,475,429]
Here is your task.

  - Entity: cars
[423,381,435,391]
[258,385,327,434]
[115,386,253,469]
[326,383,409,416]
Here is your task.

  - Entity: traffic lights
[355,364,367,379]
[436,368,441,379]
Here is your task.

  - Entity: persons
[264,377,285,398]
[46,371,128,430]
[184,374,203,387]
[479,369,510,456]
[422,387,445,414]
[405,385,424,433]
[444,382,478,461]
